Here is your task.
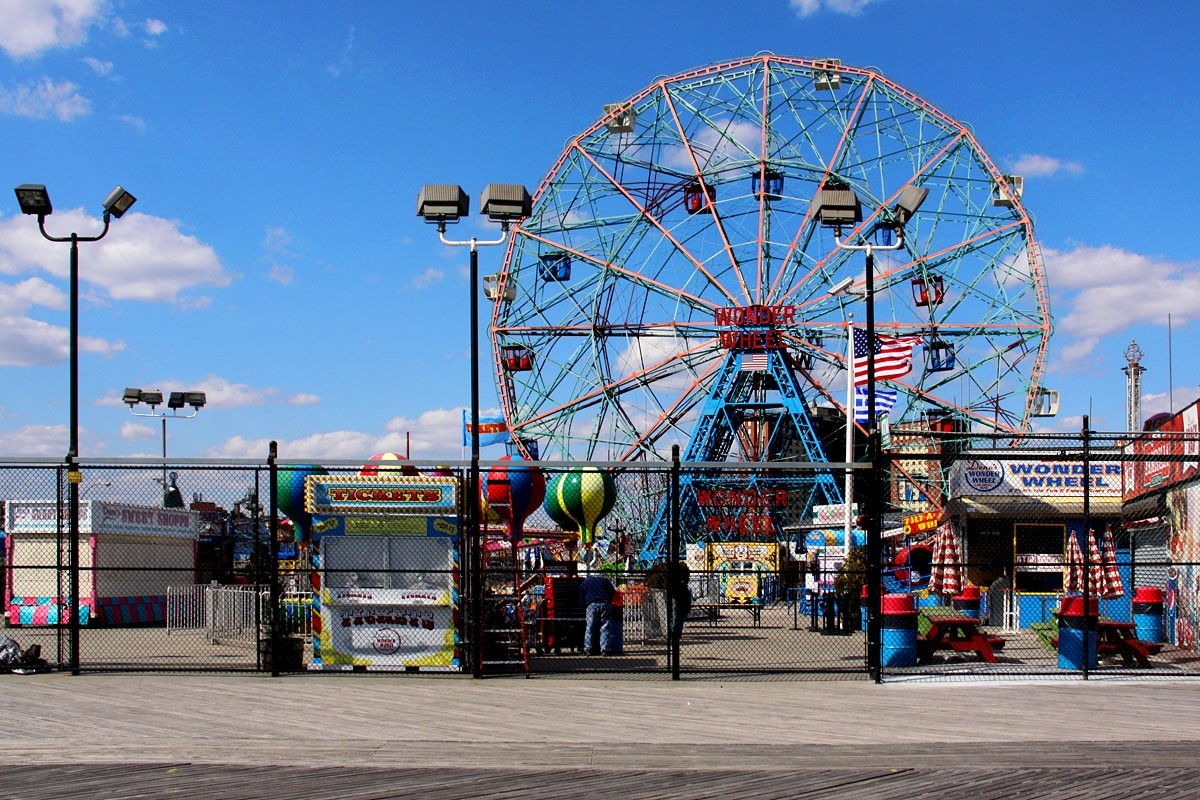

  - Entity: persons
[608,532,632,556]
[584,547,604,568]
[579,576,616,655]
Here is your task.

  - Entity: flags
[854,386,898,425]
[852,328,925,387]
[462,409,510,448]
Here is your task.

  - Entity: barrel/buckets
[881,615,917,666]
[1058,616,1097,670]
[954,601,980,619]
[1132,604,1163,642]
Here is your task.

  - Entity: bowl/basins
[860,583,887,597]
[952,585,981,601]
[1132,586,1167,604]
[881,593,919,615]
[1058,595,1100,616]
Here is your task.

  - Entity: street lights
[416,183,534,680]
[16,184,138,677]
[809,184,931,684]
[120,387,207,494]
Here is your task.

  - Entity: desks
[1034,608,1160,669]
[919,606,1005,665]
[536,617,586,656]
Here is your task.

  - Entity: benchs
[985,634,1005,643]
[691,603,764,627]
[1138,640,1164,647]
[1031,623,1056,637]
[1051,637,1059,646]
[918,636,927,641]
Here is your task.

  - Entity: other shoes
[585,649,591,656]
[602,650,608,656]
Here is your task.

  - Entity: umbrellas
[928,519,966,612]
[1060,528,1124,617]
